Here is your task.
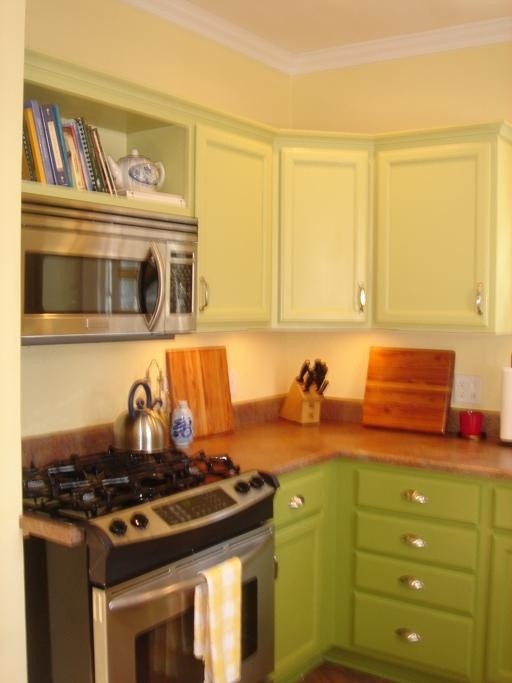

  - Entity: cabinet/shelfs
[23,50,198,227]
[272,457,338,682]
[485,476,512,682]
[376,121,511,338]
[338,457,492,683]
[275,126,376,333]
[195,105,275,331]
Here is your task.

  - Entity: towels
[194,555,242,683]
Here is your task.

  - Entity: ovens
[95,525,274,682]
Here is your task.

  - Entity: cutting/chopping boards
[361,345,455,435]
[167,346,236,439]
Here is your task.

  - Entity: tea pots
[108,148,165,194]
[112,379,169,455]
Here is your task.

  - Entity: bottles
[170,400,195,451]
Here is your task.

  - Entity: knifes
[298,360,329,395]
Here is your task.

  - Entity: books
[22,98,119,197]
[118,188,186,207]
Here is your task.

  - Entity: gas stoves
[21,444,258,521]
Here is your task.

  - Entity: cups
[459,412,484,439]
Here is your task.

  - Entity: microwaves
[22,194,198,341]
